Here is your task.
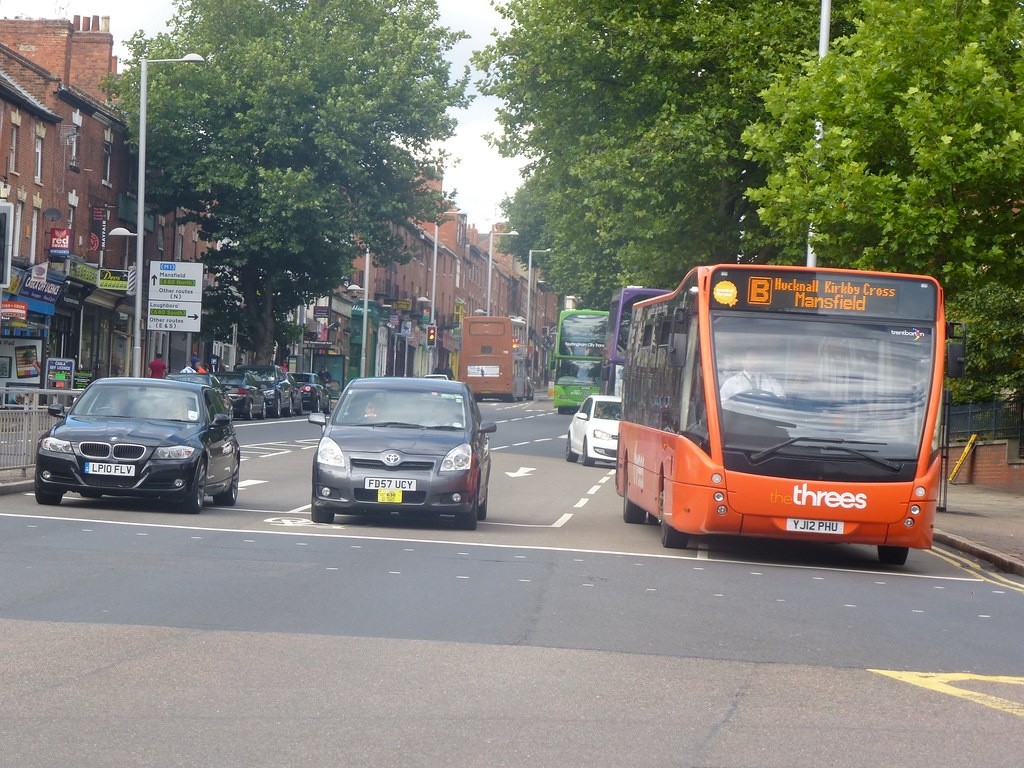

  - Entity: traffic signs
[146,258,203,334]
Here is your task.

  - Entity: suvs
[287,371,332,414]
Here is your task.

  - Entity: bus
[550,307,610,414]
[615,264,966,567]
[600,286,674,395]
[548,327,559,398]
[460,315,529,402]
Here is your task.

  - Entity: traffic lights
[427,325,437,347]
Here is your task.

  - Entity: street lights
[348,284,368,378]
[476,231,519,314]
[416,209,469,374]
[107,51,204,375]
[517,248,553,341]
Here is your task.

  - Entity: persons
[719,348,786,401]
[354,400,387,422]
[419,403,461,427]
[190,352,199,370]
[148,353,168,378]
[433,363,455,380]
[220,360,226,371]
[100,389,129,416]
[180,361,196,381]
[195,362,207,373]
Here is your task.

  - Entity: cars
[526,377,536,401]
[565,397,624,466]
[235,365,294,417]
[282,371,304,417]
[215,371,268,421]
[165,374,234,420]
[309,377,497,529]
[32,377,241,514]
